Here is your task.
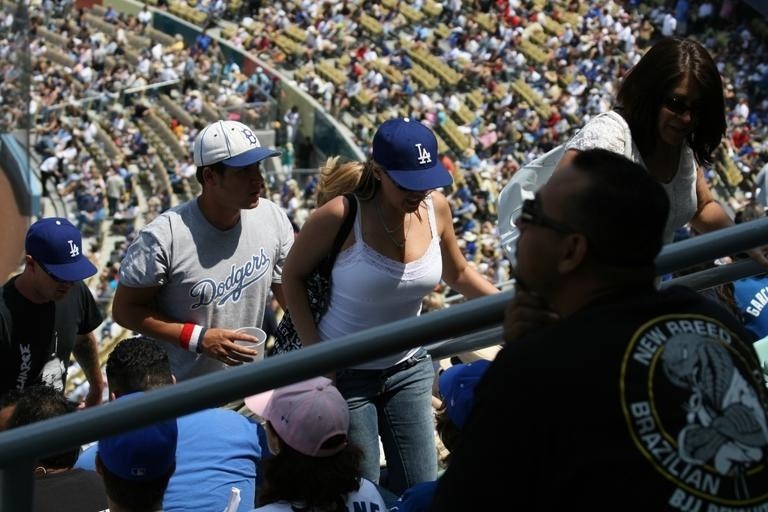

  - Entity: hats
[439,358,492,431]
[24,216,97,282]
[371,116,453,191]
[194,119,282,166]
[242,376,350,458]
[100,416,176,483]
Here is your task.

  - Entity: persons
[1,0,768,511]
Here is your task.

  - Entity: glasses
[520,199,578,237]
[668,95,703,121]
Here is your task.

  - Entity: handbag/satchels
[268,271,333,357]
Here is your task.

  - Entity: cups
[231,326,267,365]
